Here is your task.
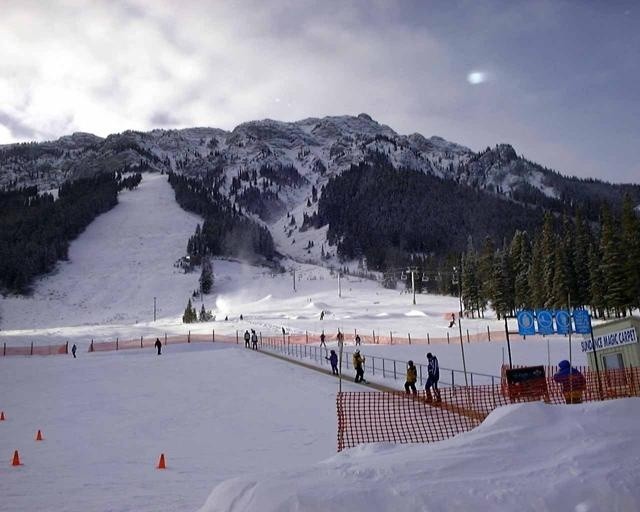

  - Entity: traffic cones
[158,453,166,469]
[0,411,4,421]
[36,429,42,441]
[11,449,21,466]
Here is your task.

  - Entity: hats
[355,348,360,354]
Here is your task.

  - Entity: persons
[325,350,339,376]
[555,360,587,406]
[337,332,344,347]
[155,338,162,355]
[244,330,251,348]
[250,330,258,351]
[319,311,324,320]
[71,343,77,358]
[425,352,442,405]
[404,360,418,395]
[448,318,456,327]
[352,348,366,383]
[319,332,326,348]
[355,334,361,346]
